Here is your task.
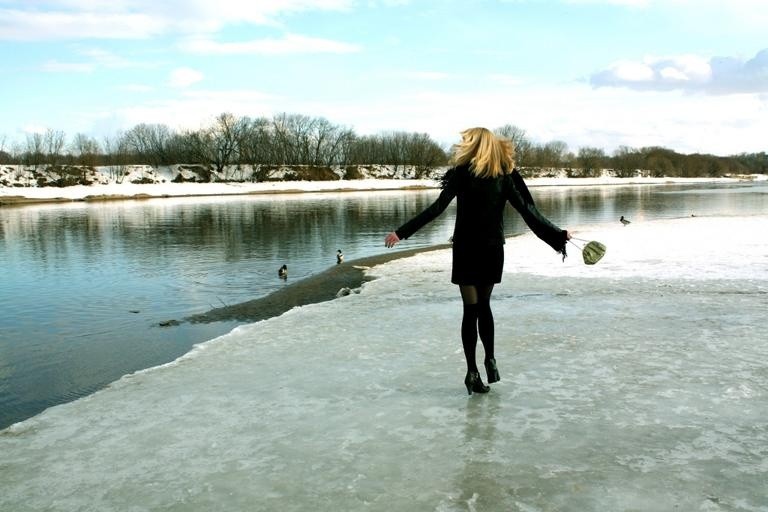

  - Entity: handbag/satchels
[568,235,607,264]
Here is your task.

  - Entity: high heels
[465,369,490,395]
[484,356,500,383]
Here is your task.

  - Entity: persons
[384,126,573,397]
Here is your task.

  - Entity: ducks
[336,249,344,261]
[620,215,631,225]
[278,264,288,277]
